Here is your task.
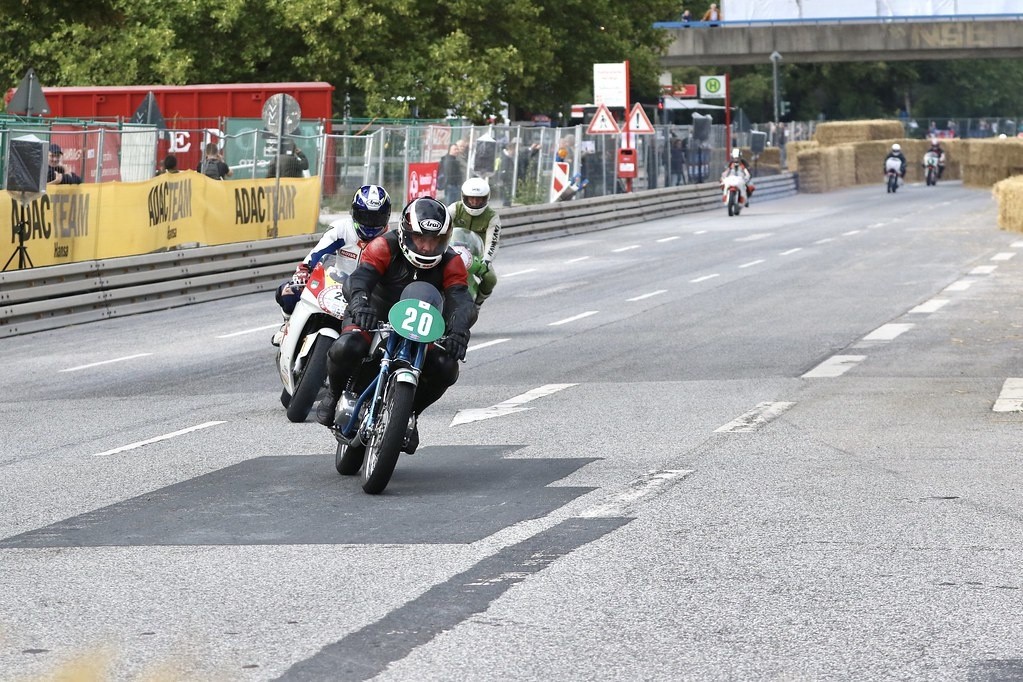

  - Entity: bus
[582,103,736,169]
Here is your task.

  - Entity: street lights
[767,51,783,132]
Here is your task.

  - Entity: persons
[558,134,575,180]
[976,120,990,130]
[437,140,467,205]
[672,138,684,186]
[929,120,960,139]
[197,143,233,180]
[928,139,946,179]
[266,147,309,178]
[883,143,907,178]
[270,185,392,348]
[499,137,541,206]
[772,122,787,169]
[316,196,478,455]
[447,177,502,309]
[156,155,179,176]
[47,144,72,185]
[1003,119,1014,137]
[648,138,660,189]
[719,149,755,208]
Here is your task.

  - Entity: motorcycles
[271,250,366,422]
[719,169,750,216]
[884,156,906,192]
[448,225,489,307]
[325,280,468,494]
[922,151,941,186]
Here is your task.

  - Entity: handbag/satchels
[436,161,447,190]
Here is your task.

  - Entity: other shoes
[271,324,288,346]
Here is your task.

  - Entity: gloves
[478,260,490,277]
[293,267,311,290]
[445,329,470,362]
[350,295,379,331]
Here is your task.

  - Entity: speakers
[7,140,43,192]
[751,133,766,153]
[693,117,711,141]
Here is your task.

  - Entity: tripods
[2,191,34,272]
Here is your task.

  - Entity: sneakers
[404,425,419,454]
[316,389,338,427]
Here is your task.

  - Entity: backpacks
[204,160,220,180]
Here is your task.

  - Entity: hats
[49,144,64,155]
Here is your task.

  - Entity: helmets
[731,148,742,159]
[932,140,940,151]
[398,196,453,269]
[351,184,392,241]
[461,178,491,216]
[891,144,901,156]
